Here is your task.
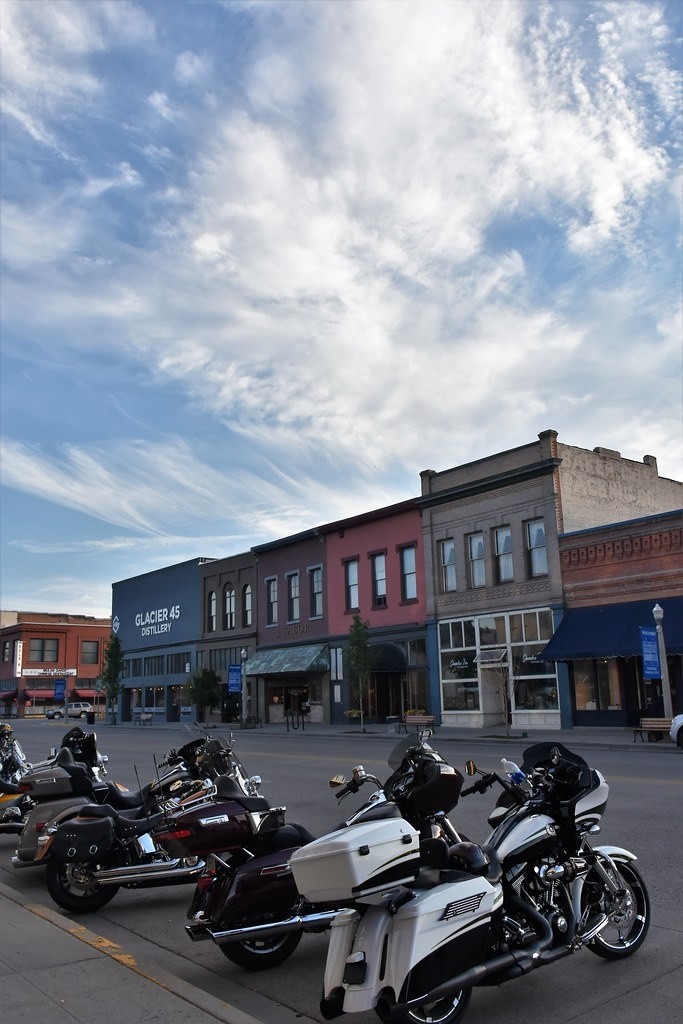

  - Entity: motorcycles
[0,719,270,912]
[288,740,651,1024]
[132,728,474,972]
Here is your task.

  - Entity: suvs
[44,701,94,720]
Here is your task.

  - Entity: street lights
[64,670,71,723]
[240,648,250,729]
[652,603,675,719]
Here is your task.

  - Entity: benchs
[133,714,153,726]
[632,718,676,743]
[398,715,442,734]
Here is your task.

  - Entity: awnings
[536,596,683,662]
[0,688,107,698]
[241,643,331,674]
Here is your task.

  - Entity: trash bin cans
[386,716,400,732]
[86,712,96,724]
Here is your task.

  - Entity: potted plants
[230,713,262,729]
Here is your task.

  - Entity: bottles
[501,759,531,793]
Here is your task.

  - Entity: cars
[669,714,683,751]
